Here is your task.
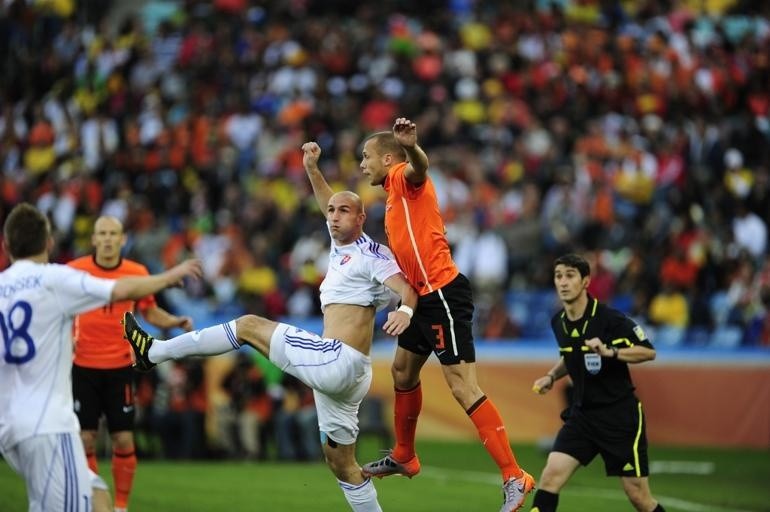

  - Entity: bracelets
[545,375,553,385]
[397,305,413,319]
[613,347,618,359]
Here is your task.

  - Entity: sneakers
[499,468,535,512]
[363,454,421,479]
[120,310,157,372]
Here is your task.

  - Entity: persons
[0,201,204,512]
[532,254,667,512]
[359,117,536,512]
[121,140,420,512]
